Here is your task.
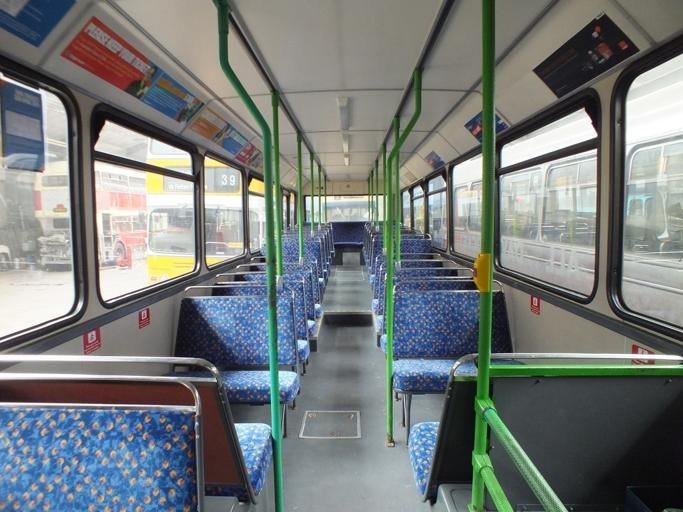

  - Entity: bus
[30,157,148,273]
[318,195,387,222]
[144,135,310,287]
[417,54,683,331]
